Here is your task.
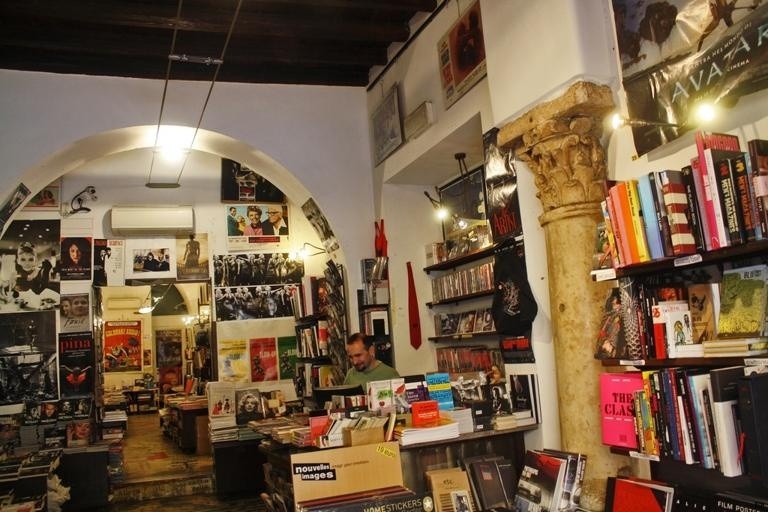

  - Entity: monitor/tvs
[313,385,363,409]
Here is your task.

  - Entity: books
[0,131,768,512]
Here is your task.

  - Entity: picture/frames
[369,81,404,168]
[439,164,484,241]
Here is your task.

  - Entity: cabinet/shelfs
[293,312,346,413]
[423,235,526,382]
[209,439,264,500]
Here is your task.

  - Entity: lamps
[180,315,200,327]
[423,192,445,220]
[69,184,99,216]
[449,211,467,229]
[612,114,681,132]
[138,305,154,314]
[287,243,310,260]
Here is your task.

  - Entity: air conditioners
[109,206,196,239]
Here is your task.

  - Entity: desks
[257,423,537,511]
[0,441,108,512]
[123,388,158,414]
[591,240,768,512]
[158,393,208,456]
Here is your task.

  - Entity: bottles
[184,365,192,393]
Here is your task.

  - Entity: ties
[407,261,422,351]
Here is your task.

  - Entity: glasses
[265,212,279,216]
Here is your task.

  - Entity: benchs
[101,411,128,439]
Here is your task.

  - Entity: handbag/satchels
[492,239,538,337]
